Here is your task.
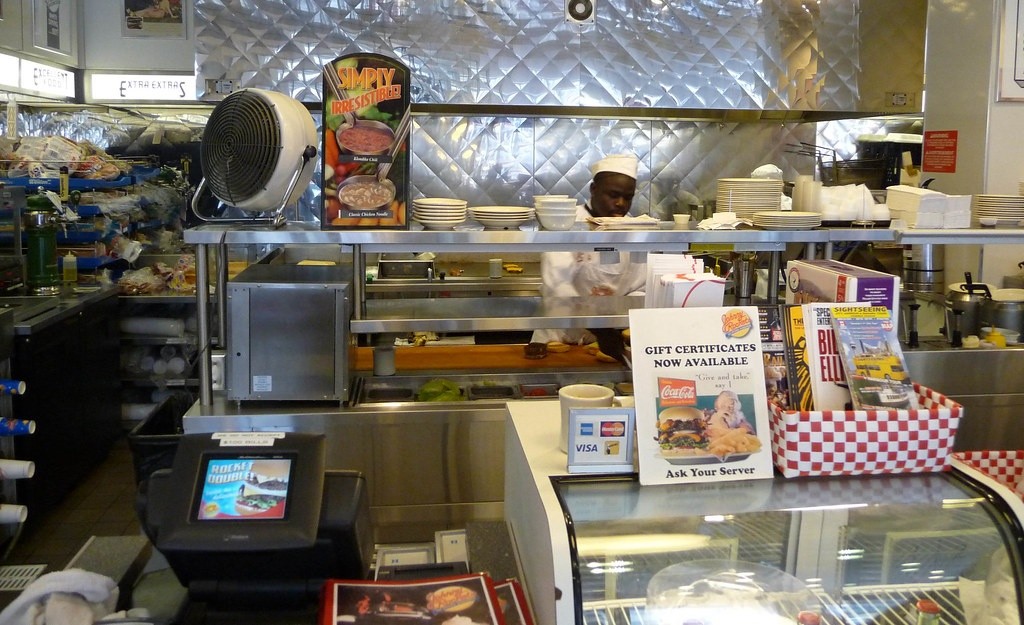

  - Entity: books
[832,306,921,410]
[782,303,815,412]
[802,302,861,412]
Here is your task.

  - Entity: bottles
[0,378,27,395]
[0,504,28,524]
[0,459,35,480]
[915,598,940,625]
[0,416,36,436]
[62,250,77,283]
[985,324,1006,350]
[797,610,820,625]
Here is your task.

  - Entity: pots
[939,281,997,348]
[897,291,916,345]
[976,288,1024,343]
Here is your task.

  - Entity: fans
[192,87,318,227]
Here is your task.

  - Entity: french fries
[708,428,761,455]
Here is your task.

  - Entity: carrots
[325,130,339,167]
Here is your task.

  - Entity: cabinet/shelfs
[0,162,222,388]
[184,221,1024,517]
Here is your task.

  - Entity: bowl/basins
[531,194,579,230]
[982,336,1019,346]
[657,221,675,230]
[672,213,691,225]
[980,326,1020,341]
[977,216,999,228]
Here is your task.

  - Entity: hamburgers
[236,460,291,515]
[657,408,710,457]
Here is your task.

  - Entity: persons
[529,157,654,350]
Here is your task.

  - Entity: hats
[589,155,638,180]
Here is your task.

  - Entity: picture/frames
[32,0,74,56]
[121,0,187,39]
[996,0,1023,102]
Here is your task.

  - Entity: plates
[468,204,536,230]
[1017,180,1024,227]
[752,210,822,232]
[972,193,1024,225]
[410,196,468,228]
[715,177,785,225]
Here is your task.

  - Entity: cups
[371,346,396,376]
[792,174,823,222]
[557,383,623,455]
[117,344,186,376]
[489,258,503,277]
[120,389,175,422]
[731,259,755,298]
[118,313,197,338]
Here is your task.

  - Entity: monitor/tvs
[192,454,295,522]
